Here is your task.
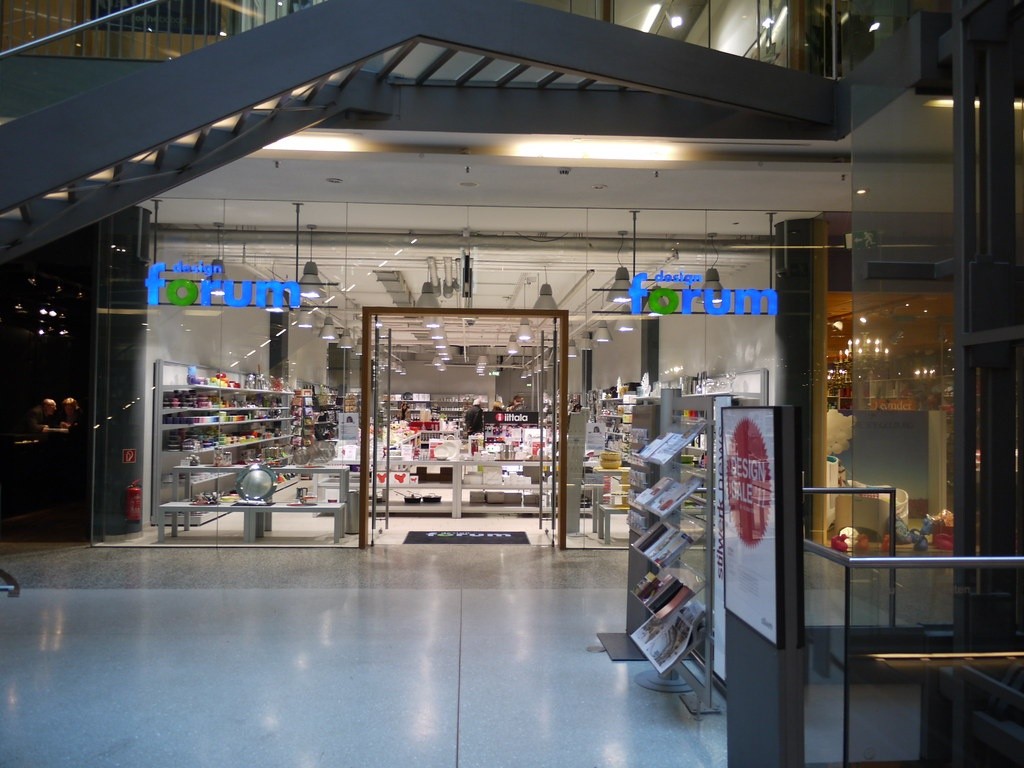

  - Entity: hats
[473,399,481,406]
[575,404,583,408]
[514,395,523,402]
[495,394,503,402]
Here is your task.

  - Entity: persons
[465,398,483,440]
[60,398,85,431]
[25,399,57,433]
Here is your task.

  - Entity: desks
[592,465,631,538]
[172,466,349,537]
[157,502,347,543]
[598,503,630,544]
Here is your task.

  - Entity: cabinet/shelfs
[317,461,458,518]
[381,395,490,422]
[314,392,338,442]
[599,397,623,455]
[151,359,299,527]
[457,460,602,520]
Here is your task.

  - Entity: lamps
[663,5,684,29]
[336,297,354,348]
[265,261,288,312]
[356,336,407,376]
[297,298,313,328]
[568,231,672,358]
[505,322,522,354]
[516,278,536,341]
[521,350,560,378]
[826,326,937,392]
[416,267,489,379]
[297,223,326,298]
[701,232,727,303]
[204,222,230,295]
[319,283,340,341]
[533,265,558,311]
